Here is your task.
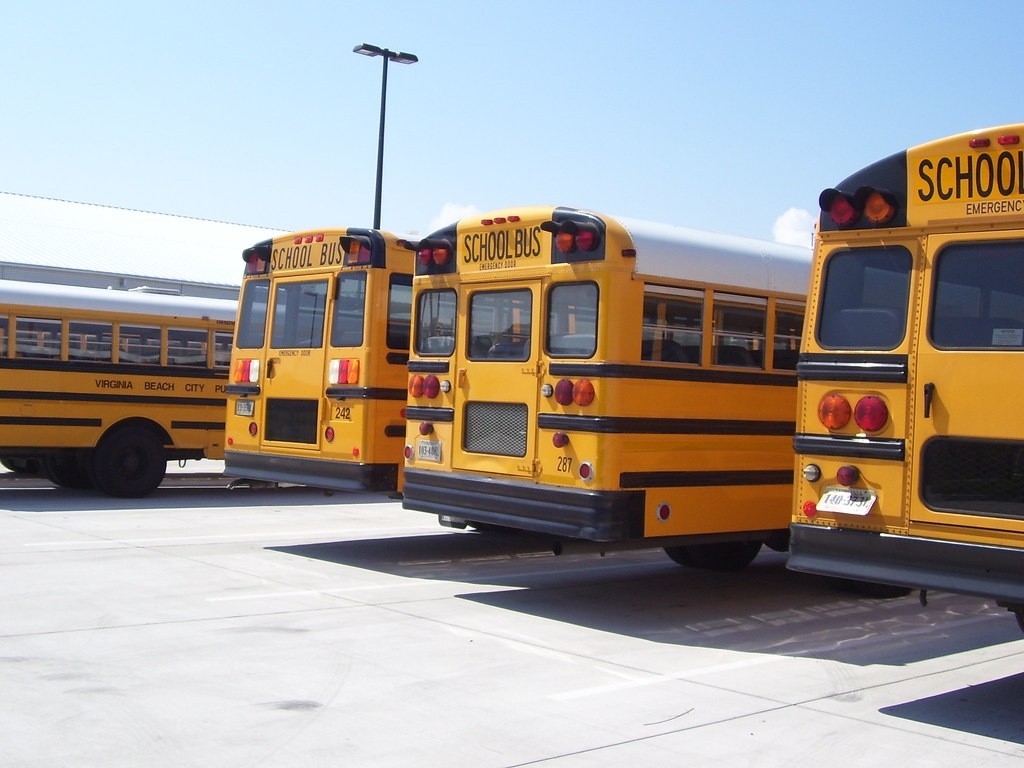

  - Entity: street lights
[353,42,419,232]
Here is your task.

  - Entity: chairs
[826,307,901,346]
[423,335,800,370]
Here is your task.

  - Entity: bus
[395,208,993,575]
[1,304,240,499]
[223,228,577,492]
[782,123,1024,606]
